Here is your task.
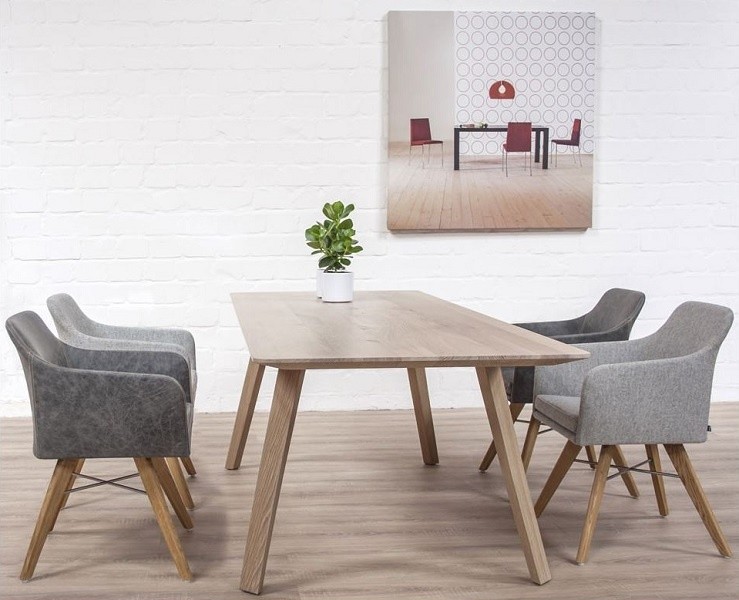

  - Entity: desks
[225,288,590,598]
[454,124,550,169]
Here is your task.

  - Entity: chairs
[6,309,196,583]
[551,118,582,169]
[501,122,533,178]
[47,292,197,510]
[533,301,734,566]
[409,118,444,170]
[478,288,646,474]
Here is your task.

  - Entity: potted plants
[304,200,364,303]
